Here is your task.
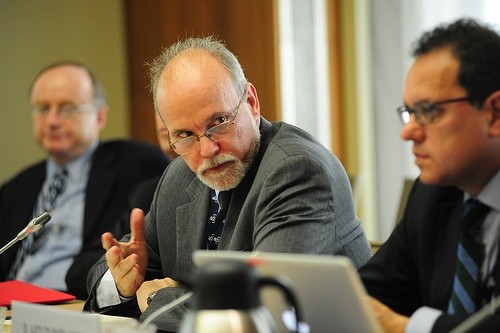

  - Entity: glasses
[397,88,489,126]
[30,101,99,121]
[168,84,248,155]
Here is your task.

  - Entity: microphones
[0,212,51,255]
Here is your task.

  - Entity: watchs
[147,291,162,304]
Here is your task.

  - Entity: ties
[448,196,493,322]
[7,168,67,280]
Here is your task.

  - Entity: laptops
[190,248,386,333]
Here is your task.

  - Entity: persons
[82,37,373,333]
[0,60,172,301]
[358,16,500,333]
